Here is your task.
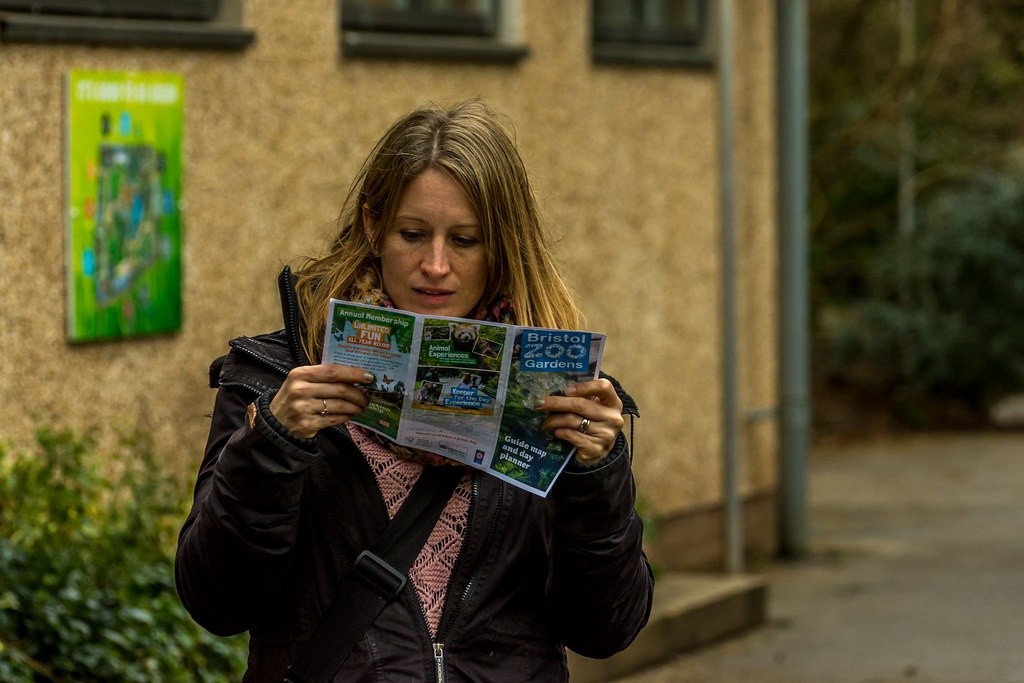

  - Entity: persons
[174,105,656,683]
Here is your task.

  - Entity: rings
[322,400,328,416]
[579,419,590,434]
